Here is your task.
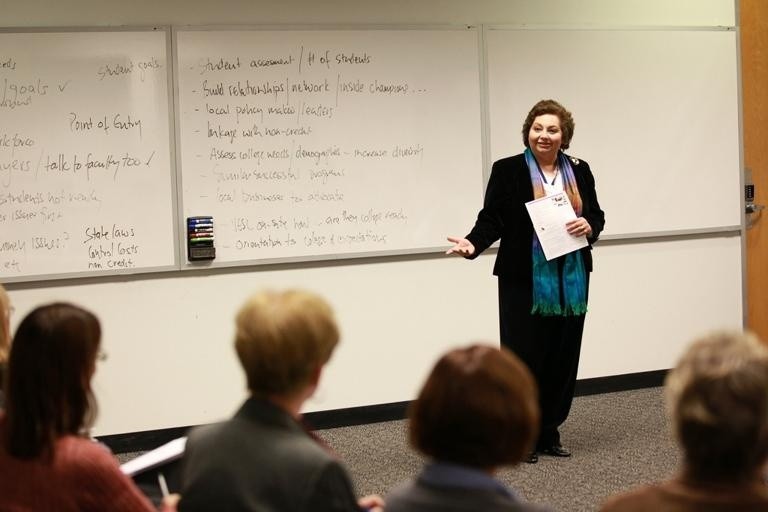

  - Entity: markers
[188,219,215,242]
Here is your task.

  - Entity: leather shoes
[524,452,539,463]
[538,445,571,457]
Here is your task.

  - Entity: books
[118,434,190,476]
[525,190,588,262]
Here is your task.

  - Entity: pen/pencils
[157,473,170,498]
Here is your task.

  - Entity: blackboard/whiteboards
[0,23,181,284]
[171,22,489,270]
[481,25,745,249]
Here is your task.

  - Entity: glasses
[7,307,13,318]
[93,352,107,364]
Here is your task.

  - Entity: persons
[442,99,606,463]
[175,285,387,512]
[597,327,766,510]
[1,300,183,512]
[378,343,560,512]
